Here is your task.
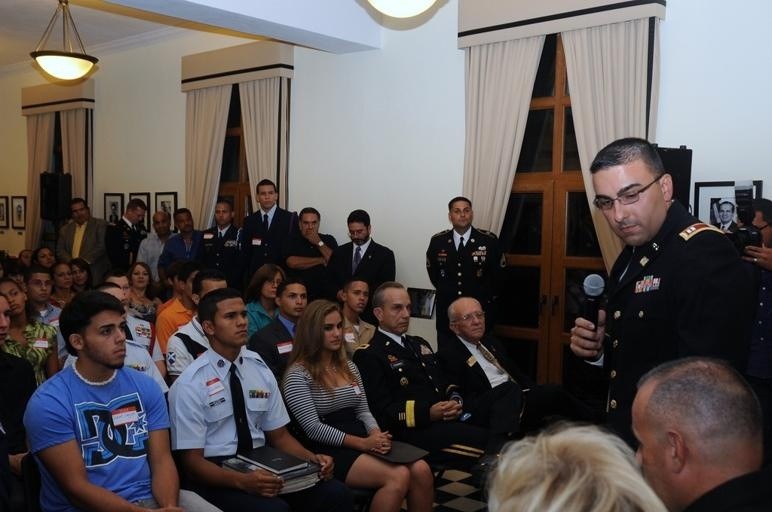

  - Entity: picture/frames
[694,180,762,232]
[104,192,124,226]
[12,195,26,228]
[129,192,150,233]
[0,196,9,229]
[155,192,177,232]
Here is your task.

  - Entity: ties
[218,231,224,246]
[399,337,417,357]
[721,226,725,230]
[458,236,465,253]
[130,225,137,237]
[229,364,253,455]
[352,246,362,276]
[263,214,270,234]
[477,343,507,376]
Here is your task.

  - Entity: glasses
[448,310,487,324]
[28,278,54,289]
[592,172,667,212]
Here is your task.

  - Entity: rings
[754,258,758,262]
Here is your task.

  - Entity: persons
[711,201,740,233]
[736,197,772,375]
[566,136,754,452]
[480,420,665,510]
[628,353,772,512]
[1,178,604,512]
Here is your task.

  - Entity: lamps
[29,0,99,81]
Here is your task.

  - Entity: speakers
[39,171,72,219]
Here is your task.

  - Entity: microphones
[582,274,605,323]
